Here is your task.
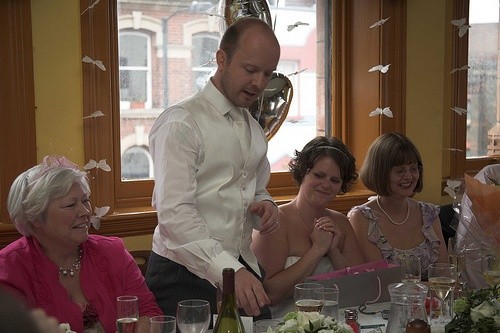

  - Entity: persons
[0,164,162,333]
[147,18,280,323]
[251,137,361,318]
[456,164,500,287]
[347,133,446,281]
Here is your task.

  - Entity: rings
[334,224,337,227]
[275,221,280,223]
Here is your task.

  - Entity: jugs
[385,277,429,333]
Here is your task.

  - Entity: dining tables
[274,295,454,333]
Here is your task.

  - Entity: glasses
[392,165,421,177]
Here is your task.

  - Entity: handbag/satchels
[302,259,401,305]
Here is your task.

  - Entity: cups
[150,299,211,333]
[116,296,140,333]
[317,288,339,322]
[295,283,325,313]
[400,256,421,284]
[481,253,500,288]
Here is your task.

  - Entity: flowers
[265,309,358,333]
[445,284,500,333]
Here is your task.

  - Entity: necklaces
[58,250,82,276]
[377,195,410,225]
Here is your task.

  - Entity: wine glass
[448,237,466,285]
[429,263,457,326]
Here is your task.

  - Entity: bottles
[214,268,246,333]
[344,309,359,333]
